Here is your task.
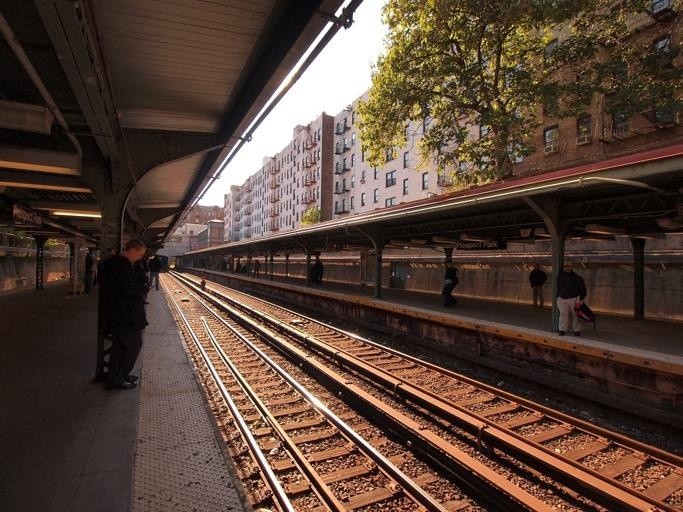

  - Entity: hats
[563,261,573,266]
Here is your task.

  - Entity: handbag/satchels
[574,299,596,323]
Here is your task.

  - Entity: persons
[253,259,261,274]
[101,240,149,390]
[311,257,323,284]
[554,261,587,337]
[83,251,95,293]
[528,263,547,308]
[106,247,161,304]
[440,261,459,307]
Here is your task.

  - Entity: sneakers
[574,330,580,336]
[558,330,567,335]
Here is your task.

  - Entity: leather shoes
[119,381,137,389]
[125,374,139,382]
